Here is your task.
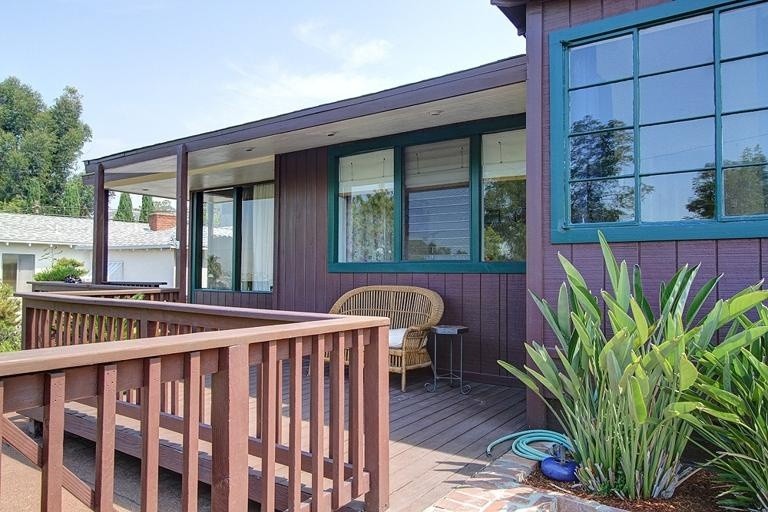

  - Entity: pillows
[388,328,428,349]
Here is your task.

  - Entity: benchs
[307,285,444,393]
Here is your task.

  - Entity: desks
[425,324,472,396]
